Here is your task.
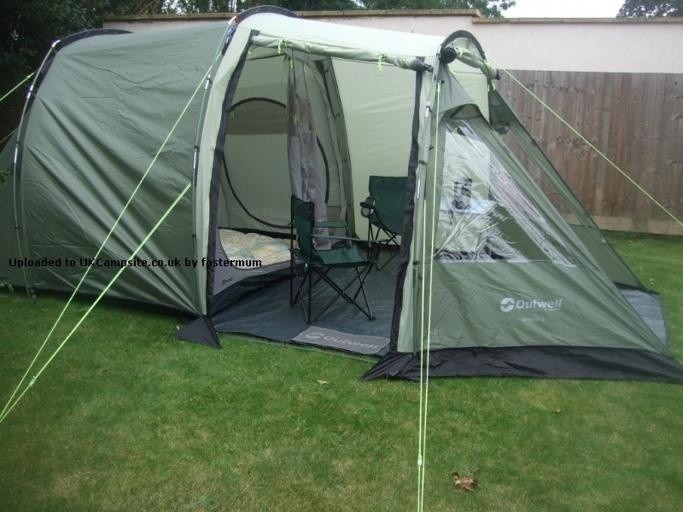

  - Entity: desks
[434,198,498,260]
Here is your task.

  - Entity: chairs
[360,177,408,271]
[289,194,378,325]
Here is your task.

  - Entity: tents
[0,5,683,385]
